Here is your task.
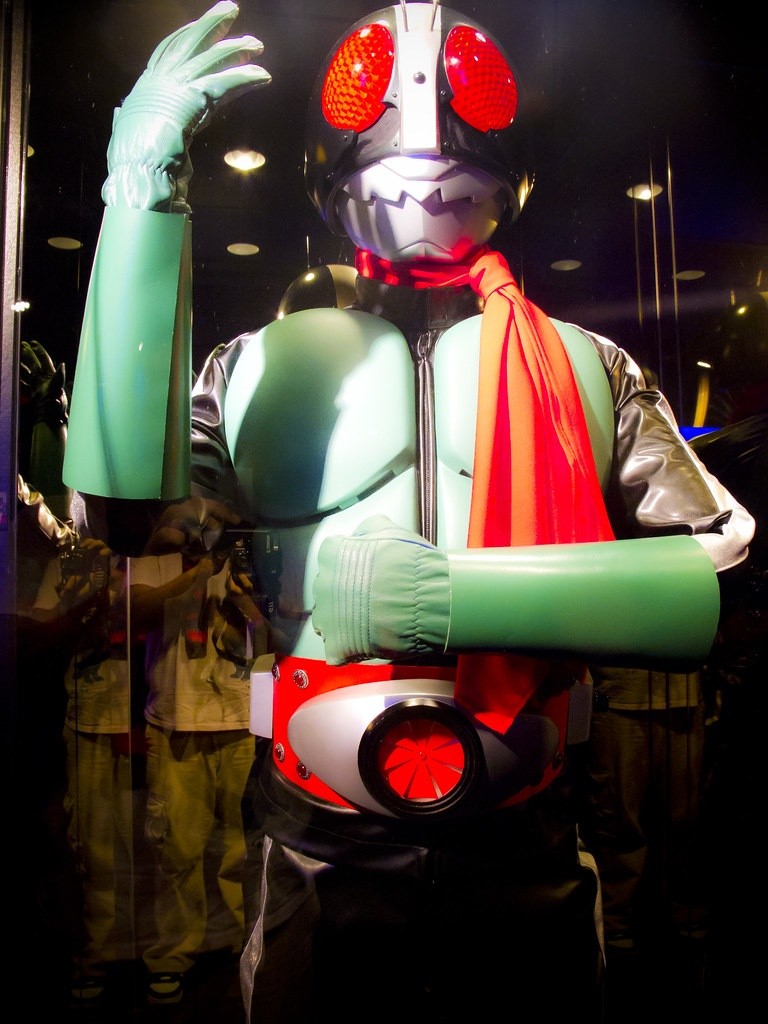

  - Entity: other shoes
[145,968,185,1008]
[70,972,102,1005]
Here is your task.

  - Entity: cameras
[226,527,284,620]
[58,550,110,618]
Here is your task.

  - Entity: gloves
[100,0,273,209]
[312,515,448,664]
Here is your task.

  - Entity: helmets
[309,0,539,264]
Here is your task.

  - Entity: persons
[60,2,753,1024]
[34,499,306,1009]
